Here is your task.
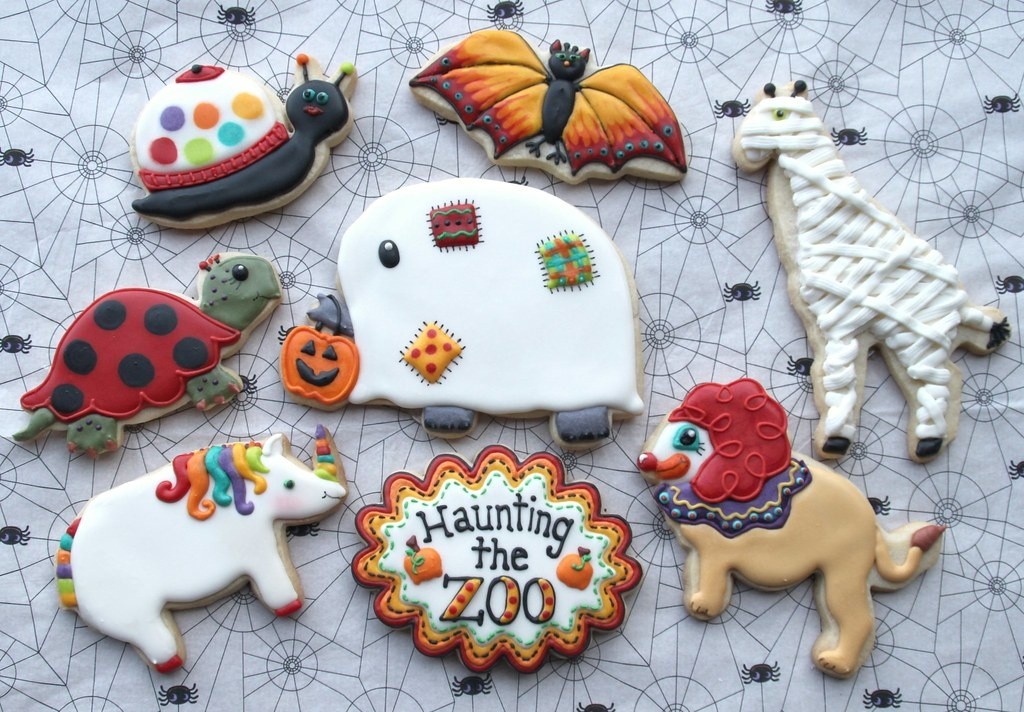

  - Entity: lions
[638,377,947,681]
